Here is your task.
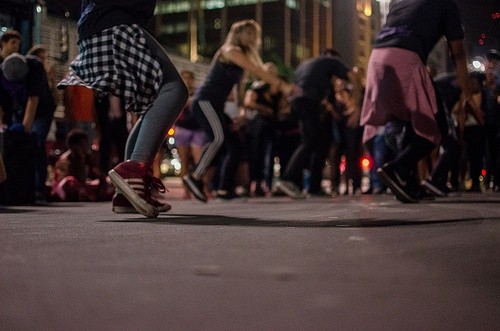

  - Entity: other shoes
[276,180,304,198]
[32,191,48,206]
[419,176,464,198]
[307,183,389,199]
[210,181,281,199]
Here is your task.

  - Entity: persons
[182,18,304,203]
[55,0,190,220]
[0,0,500,201]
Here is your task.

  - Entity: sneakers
[108,160,171,218]
[183,174,207,202]
[376,163,426,203]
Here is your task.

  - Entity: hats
[1,53,30,81]
